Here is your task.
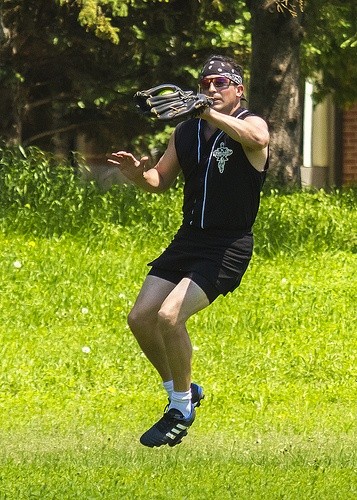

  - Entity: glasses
[199,76,238,89]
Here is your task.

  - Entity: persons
[107,56,271,449]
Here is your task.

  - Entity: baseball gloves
[134,84,215,123]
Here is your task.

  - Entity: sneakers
[139,404,197,448]
[164,383,205,447]
